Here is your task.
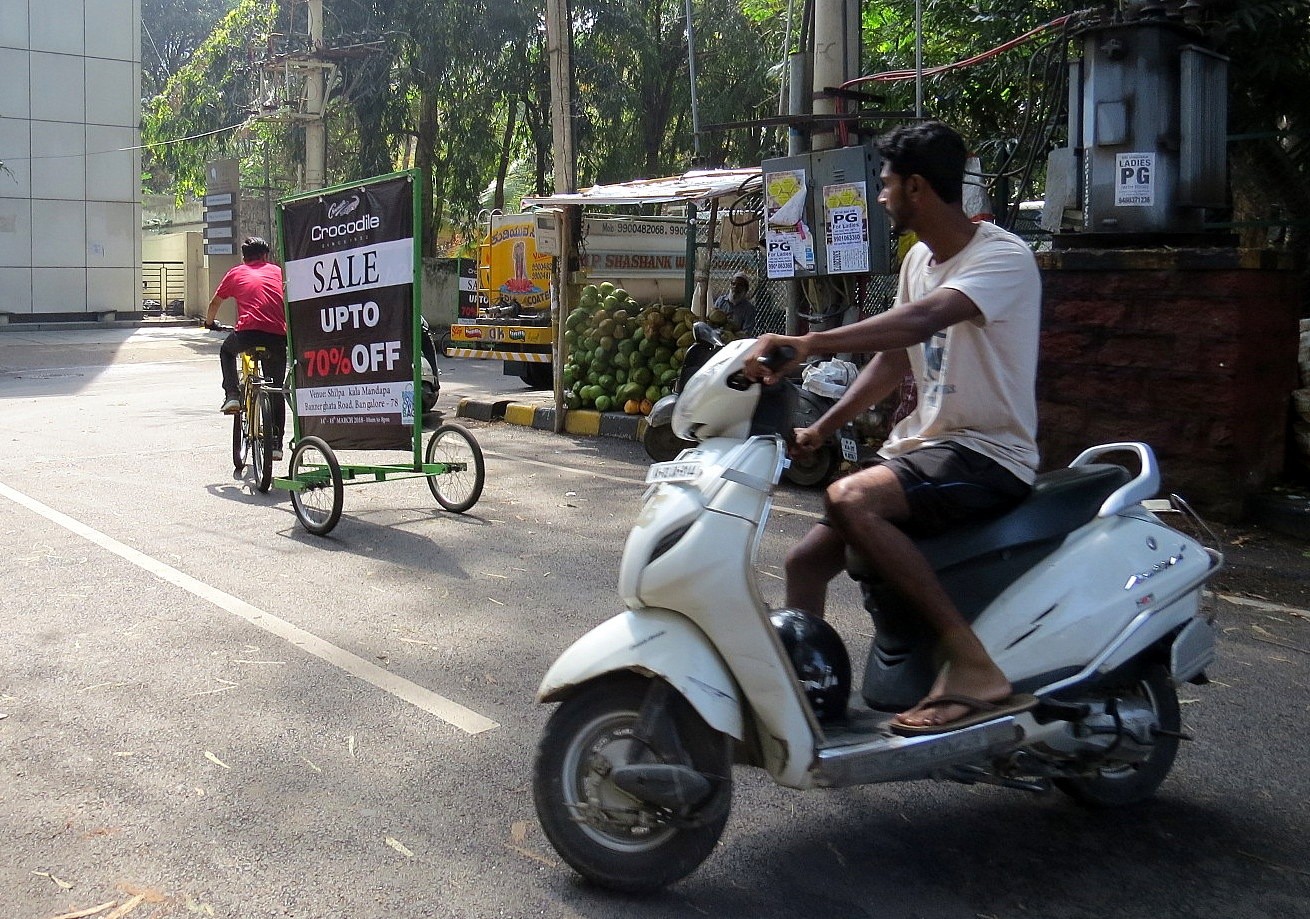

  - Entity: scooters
[642,327,861,492]
[531,322,1223,893]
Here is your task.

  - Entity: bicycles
[207,324,277,493]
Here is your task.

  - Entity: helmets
[765,609,851,726]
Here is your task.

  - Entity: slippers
[889,691,1038,734]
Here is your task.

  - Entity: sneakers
[220,394,240,411]
[271,442,282,459]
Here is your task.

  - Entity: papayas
[563,281,748,416]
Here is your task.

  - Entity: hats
[733,272,749,282]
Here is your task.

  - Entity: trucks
[447,206,761,389]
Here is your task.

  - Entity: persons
[714,272,755,337]
[744,120,1042,738]
[204,237,288,461]
[420,315,440,414]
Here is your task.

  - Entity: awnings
[520,167,763,434]
